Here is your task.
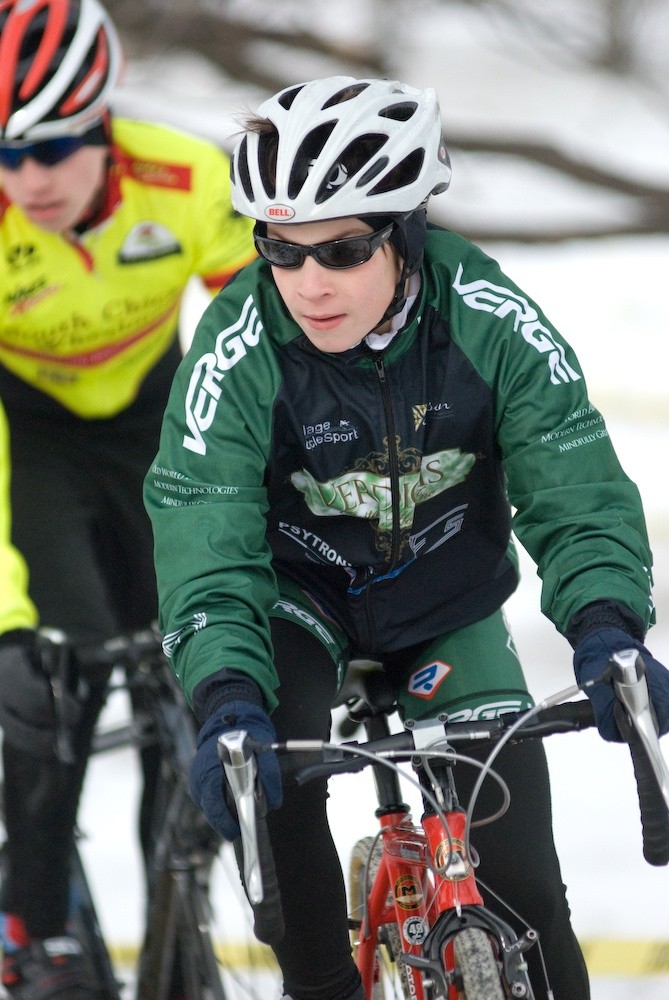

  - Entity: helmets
[0,1,126,151]
[229,76,452,224]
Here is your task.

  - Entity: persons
[143,72,668,1000]
[0,0,259,1000]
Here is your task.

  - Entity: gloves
[0,645,55,754]
[574,623,669,741]
[184,698,283,841]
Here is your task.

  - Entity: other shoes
[0,845,100,999]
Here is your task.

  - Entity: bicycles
[214,647,669,1000]
[69,623,232,999]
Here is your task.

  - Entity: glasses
[1,131,98,170]
[253,203,426,270]
